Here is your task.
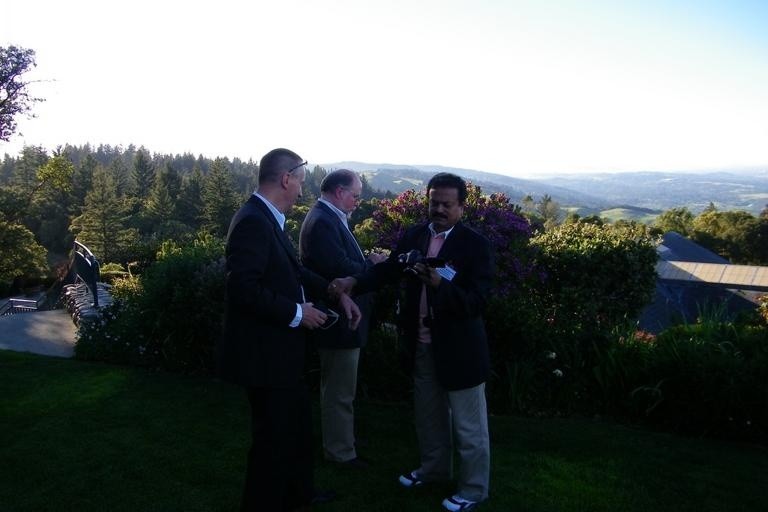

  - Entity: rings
[329,284,336,290]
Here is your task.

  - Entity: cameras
[399,248,444,279]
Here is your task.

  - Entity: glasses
[288,160,307,172]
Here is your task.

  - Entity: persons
[298,169,390,471]
[326,171,497,510]
[222,146,363,509]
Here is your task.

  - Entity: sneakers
[442,495,478,512]
[399,471,424,487]
